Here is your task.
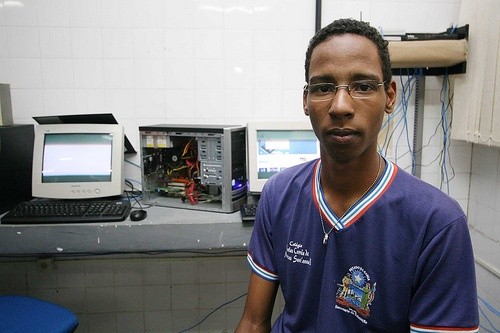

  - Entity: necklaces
[317,152,382,243]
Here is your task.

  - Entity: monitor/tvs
[245,119,322,194]
[31,125,125,199]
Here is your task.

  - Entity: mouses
[129,210,147,221]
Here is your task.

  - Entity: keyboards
[239,203,259,222]
[0,200,132,225]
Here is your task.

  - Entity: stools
[0,294,79,333]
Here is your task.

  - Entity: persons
[234,19,480,333]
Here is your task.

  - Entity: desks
[0,195,285,333]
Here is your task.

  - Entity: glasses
[306,79,388,102]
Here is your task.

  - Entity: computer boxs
[138,122,248,214]
